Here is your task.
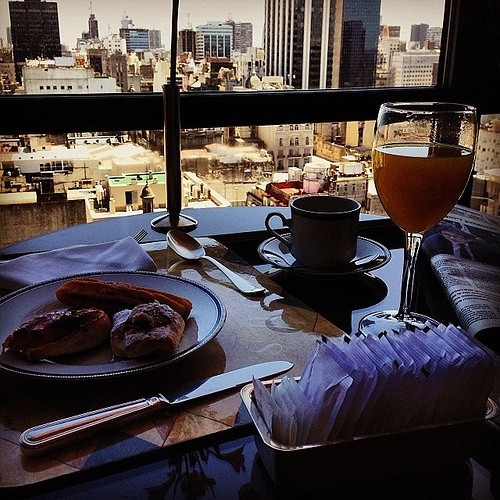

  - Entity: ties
[459,244,472,260]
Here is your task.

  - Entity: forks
[134,228,147,243]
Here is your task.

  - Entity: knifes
[17,361,293,453]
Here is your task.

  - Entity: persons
[424,223,494,266]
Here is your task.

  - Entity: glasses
[442,233,463,241]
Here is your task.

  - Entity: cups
[265,196,361,268]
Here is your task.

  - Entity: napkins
[0,236,159,286]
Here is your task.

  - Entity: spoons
[350,252,381,269]
[165,229,265,298]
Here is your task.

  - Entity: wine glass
[358,102,480,342]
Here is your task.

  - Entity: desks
[0,204,500,500]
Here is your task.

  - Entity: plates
[0,270,227,381]
[257,233,391,276]
[240,374,499,452]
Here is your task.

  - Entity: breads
[1,278,193,359]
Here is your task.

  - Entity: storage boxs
[238,374,498,481]
